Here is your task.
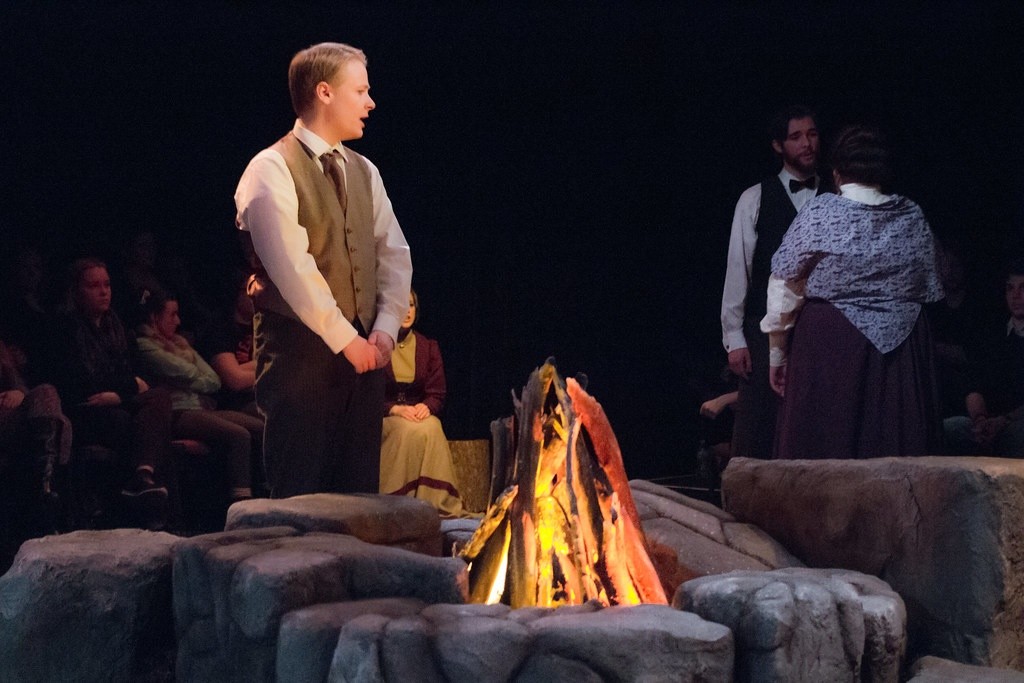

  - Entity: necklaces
[390,334,414,349]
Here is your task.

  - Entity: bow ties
[789,176,815,193]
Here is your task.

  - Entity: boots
[31,432,58,499]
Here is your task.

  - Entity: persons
[758,123,953,486]
[210,264,258,502]
[136,289,267,501]
[66,258,170,505]
[943,267,1024,458]
[232,40,415,499]
[0,298,75,522]
[700,109,846,467]
[378,287,465,511]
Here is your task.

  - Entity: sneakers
[121,470,167,505]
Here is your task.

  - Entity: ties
[321,152,348,212]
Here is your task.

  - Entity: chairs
[69,437,212,468]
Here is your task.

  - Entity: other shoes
[228,477,251,499]
